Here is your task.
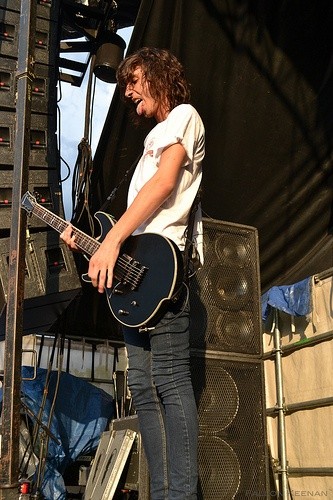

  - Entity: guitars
[20,189,185,330]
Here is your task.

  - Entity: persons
[58,48,206,500]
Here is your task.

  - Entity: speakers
[189,353,270,500]
[189,218,264,359]
